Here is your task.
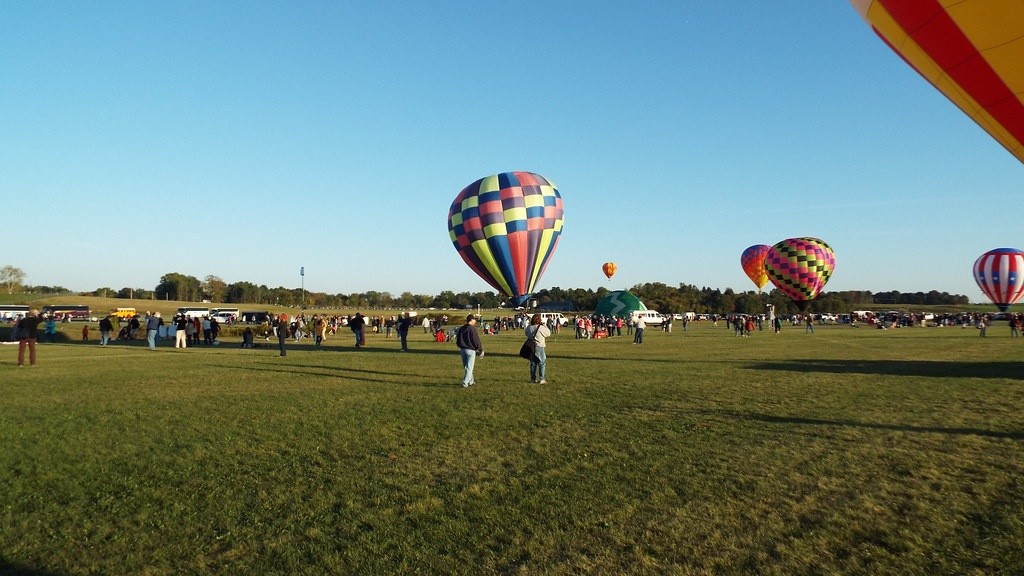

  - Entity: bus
[0,305,30,321]
[210,308,240,317]
[39,305,89,320]
[110,307,136,317]
[177,308,209,319]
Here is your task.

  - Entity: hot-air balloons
[448,171,565,326]
[764,236,835,319]
[973,248,1024,319]
[601,261,618,282]
[741,244,771,295]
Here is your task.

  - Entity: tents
[595,291,648,319]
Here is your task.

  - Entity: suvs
[209,312,237,324]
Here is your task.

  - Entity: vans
[536,312,568,327]
[240,311,267,324]
[631,310,664,327]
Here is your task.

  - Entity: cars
[660,313,682,320]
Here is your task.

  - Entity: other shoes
[463,385,468,387]
[539,379,546,385]
[532,380,537,382]
[468,380,476,385]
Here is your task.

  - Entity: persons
[128,311,221,348]
[775,316,781,333]
[713,314,763,338]
[83,325,89,341]
[547,315,560,333]
[372,312,449,351]
[457,314,484,387]
[1009,313,1024,337]
[99,316,114,345]
[525,315,551,384]
[11,309,55,367]
[228,313,366,356]
[684,316,688,331]
[662,317,672,333]
[934,313,990,337]
[841,313,925,328]
[572,315,645,344]
[794,315,814,334]
[479,314,529,335]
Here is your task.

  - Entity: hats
[467,314,478,319]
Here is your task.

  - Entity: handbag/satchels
[519,339,536,360]
